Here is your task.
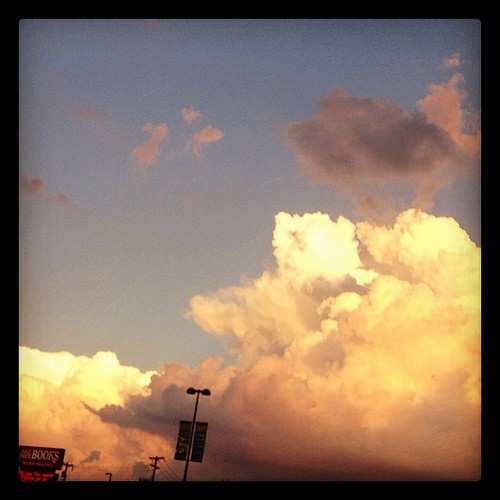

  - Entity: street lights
[105,473,112,481]
[182,387,211,479]
